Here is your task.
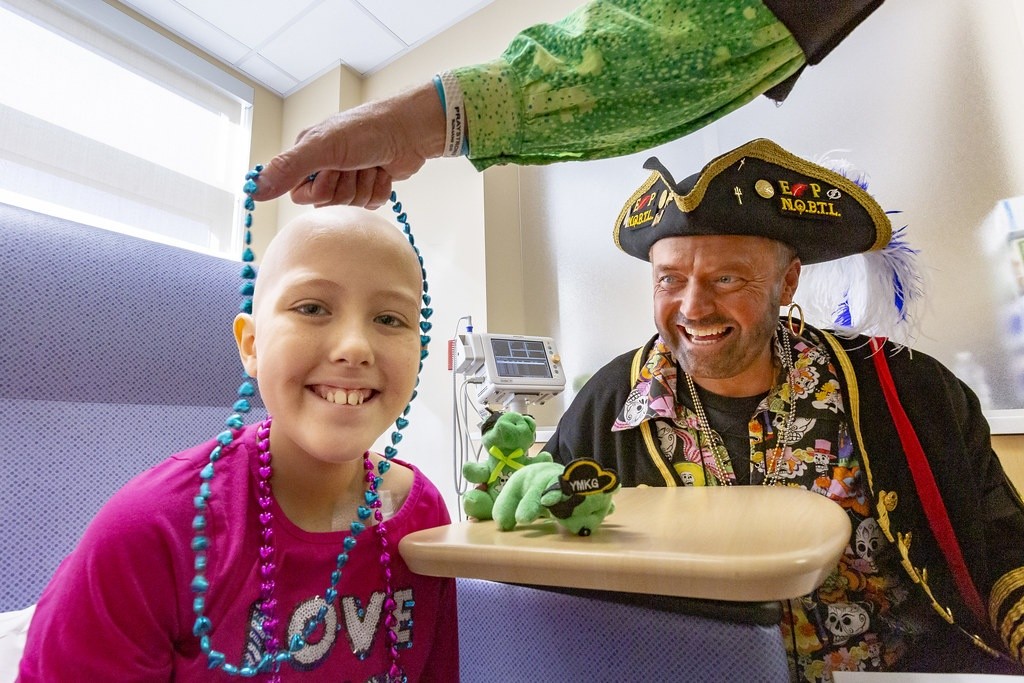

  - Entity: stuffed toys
[462,411,554,520]
[492,460,620,538]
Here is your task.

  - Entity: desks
[396,487,852,683]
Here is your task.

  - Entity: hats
[611,136,893,265]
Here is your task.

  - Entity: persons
[529,177,1024,683]
[18,204,461,682]
[250,0,887,209]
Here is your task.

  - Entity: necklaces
[259,416,402,682]
[685,318,795,486]
[191,160,432,678]
